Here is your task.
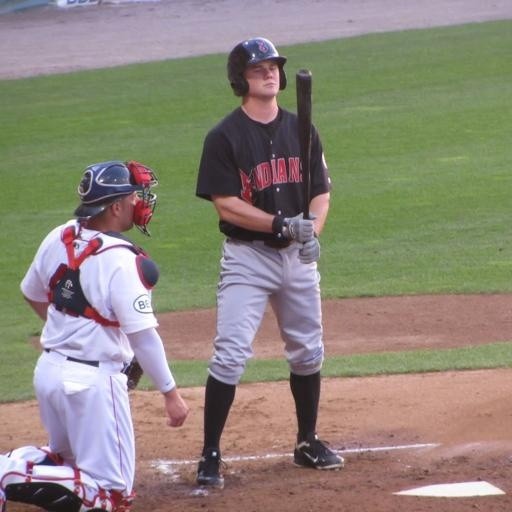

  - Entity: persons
[0,162,190,512]
[195,38,344,489]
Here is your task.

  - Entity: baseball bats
[296,68,312,218]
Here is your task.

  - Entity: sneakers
[197,446,229,489]
[292,432,345,470]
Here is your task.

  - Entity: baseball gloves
[124,357,142,389]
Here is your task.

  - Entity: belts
[44,348,99,367]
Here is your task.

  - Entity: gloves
[298,237,321,264]
[281,211,317,243]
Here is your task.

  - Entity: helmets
[75,161,159,237]
[228,37,287,97]
